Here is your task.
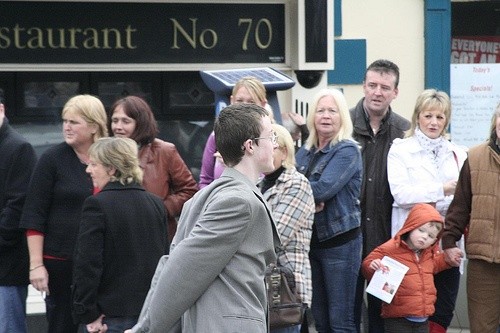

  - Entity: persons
[198,75,274,191]
[293,88,367,333]
[346,59,414,332]
[384,89,469,332]
[442,103,500,333]
[357,200,465,333]
[256,124,315,333]
[19,93,110,333]
[100,94,199,245]
[71,137,166,332]
[122,104,282,333]
[0,91,36,333]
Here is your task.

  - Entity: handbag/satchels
[264,264,306,329]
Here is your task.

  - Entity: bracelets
[29,265,45,272]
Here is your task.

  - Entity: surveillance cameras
[294,71,324,89]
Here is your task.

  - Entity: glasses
[244,132,277,144]
